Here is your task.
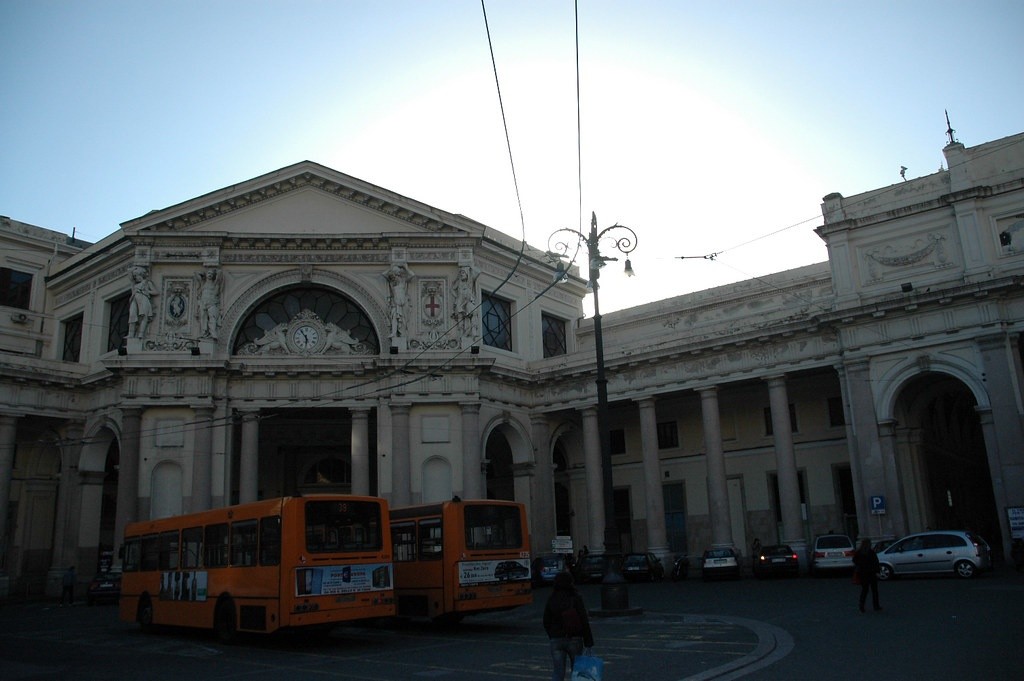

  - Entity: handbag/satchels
[852,576,860,584]
[570,644,603,681]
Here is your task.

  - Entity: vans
[812,535,854,577]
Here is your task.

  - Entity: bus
[115,489,528,642]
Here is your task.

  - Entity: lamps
[472,346,479,354]
[901,282,913,292]
[191,347,200,356]
[390,346,398,354]
[118,348,126,355]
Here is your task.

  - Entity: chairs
[466,541,511,549]
[308,544,377,552]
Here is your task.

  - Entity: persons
[751,538,762,558]
[192,268,224,341]
[543,571,595,681]
[124,267,160,339]
[852,538,882,613]
[451,267,482,337]
[58,566,76,609]
[575,544,589,569]
[382,261,415,338]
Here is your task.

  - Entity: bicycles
[672,553,684,578]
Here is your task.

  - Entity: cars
[620,552,664,584]
[700,547,740,582]
[876,530,991,579]
[762,545,801,579]
[531,550,622,587]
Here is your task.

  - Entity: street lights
[543,210,637,613]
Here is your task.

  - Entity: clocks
[286,309,326,353]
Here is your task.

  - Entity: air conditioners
[11,311,28,323]
